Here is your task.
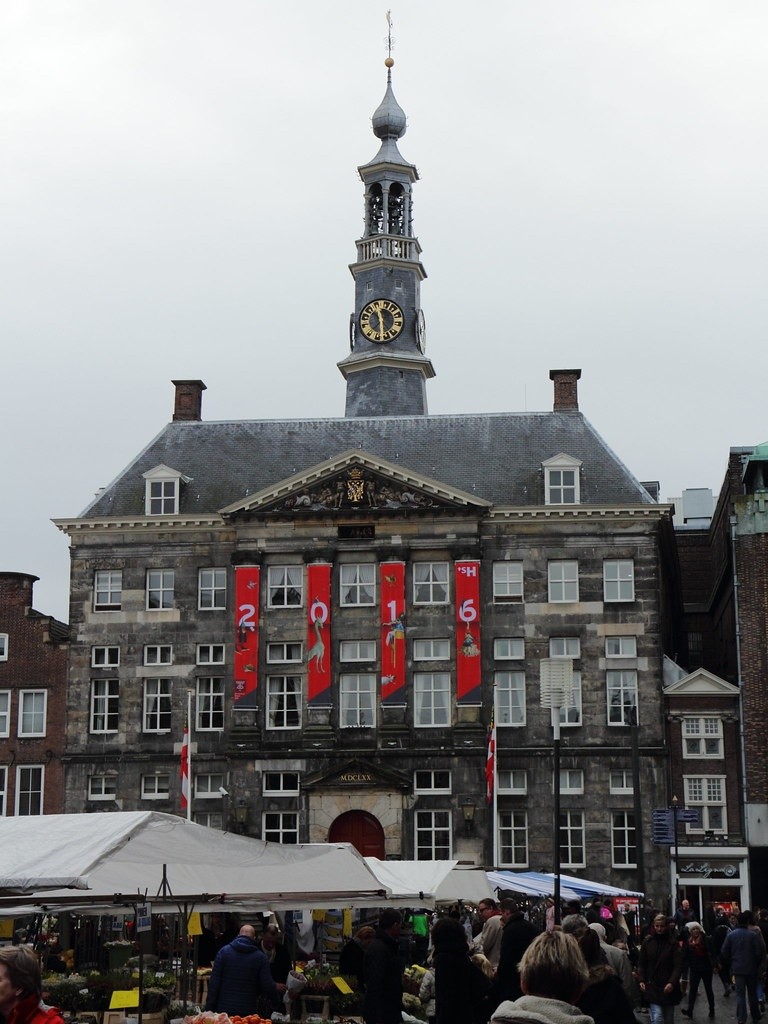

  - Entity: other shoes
[759,999,765,1012]
[724,992,729,997]
[709,1012,715,1017]
[731,986,735,991]
[753,1015,761,1023]
[738,1019,746,1024]
[681,1009,692,1017]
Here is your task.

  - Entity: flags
[484,704,494,804]
[179,707,188,809]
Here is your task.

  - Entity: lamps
[234,798,251,836]
[461,797,476,837]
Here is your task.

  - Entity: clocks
[416,308,426,355]
[350,313,355,352]
[359,298,405,344]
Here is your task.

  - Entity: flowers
[283,970,307,1003]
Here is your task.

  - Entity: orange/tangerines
[230,1014,272,1024]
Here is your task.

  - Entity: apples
[192,1012,233,1024]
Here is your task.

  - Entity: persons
[0,943,67,1024]
[721,914,766,1024]
[332,476,346,508]
[703,899,768,1013]
[398,898,681,1024]
[365,476,378,507]
[673,899,696,929]
[339,908,403,1024]
[380,483,392,497]
[681,921,717,1018]
[588,922,632,998]
[318,483,332,503]
[497,898,538,1005]
[638,914,683,1024]
[620,902,635,949]
[205,926,293,1016]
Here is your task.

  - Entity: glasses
[476,907,491,913]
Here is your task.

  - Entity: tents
[363,856,500,910]
[0,811,392,911]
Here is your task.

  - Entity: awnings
[487,870,645,902]
